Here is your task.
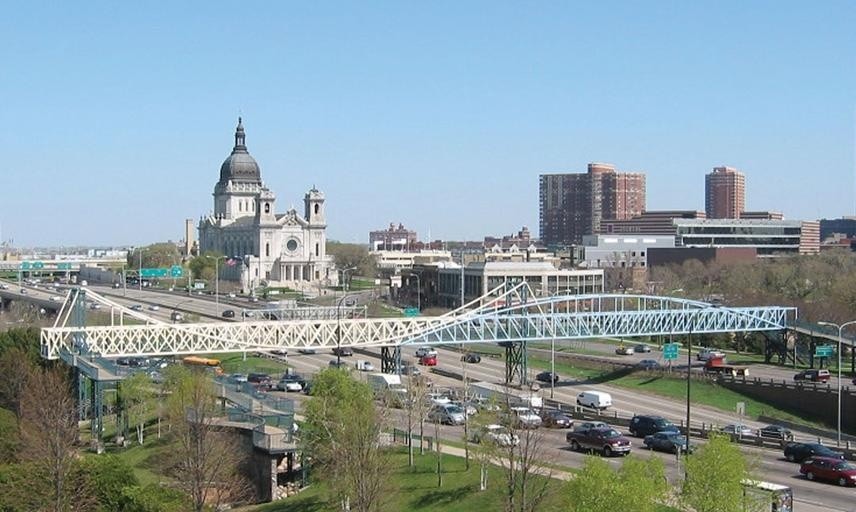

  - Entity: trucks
[577,390,612,411]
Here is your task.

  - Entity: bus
[184,356,224,379]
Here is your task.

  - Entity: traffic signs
[141,265,183,278]
[22,261,44,269]
[57,263,72,270]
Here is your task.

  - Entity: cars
[424,391,574,447]
[567,421,633,457]
[171,313,182,320]
[21,278,88,301]
[242,312,253,317]
[262,313,277,320]
[112,282,119,288]
[723,425,794,441]
[629,415,696,455]
[90,303,101,310]
[784,443,856,487]
[330,346,438,409]
[230,373,305,399]
[222,311,234,317]
[461,355,481,363]
[0,284,9,290]
[794,368,830,383]
[616,345,659,367]
[133,304,160,311]
[537,372,558,383]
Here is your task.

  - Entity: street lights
[668,289,683,374]
[817,321,856,447]
[139,248,150,291]
[343,266,357,314]
[410,273,420,310]
[174,300,193,324]
[206,256,228,317]
[535,289,572,398]
